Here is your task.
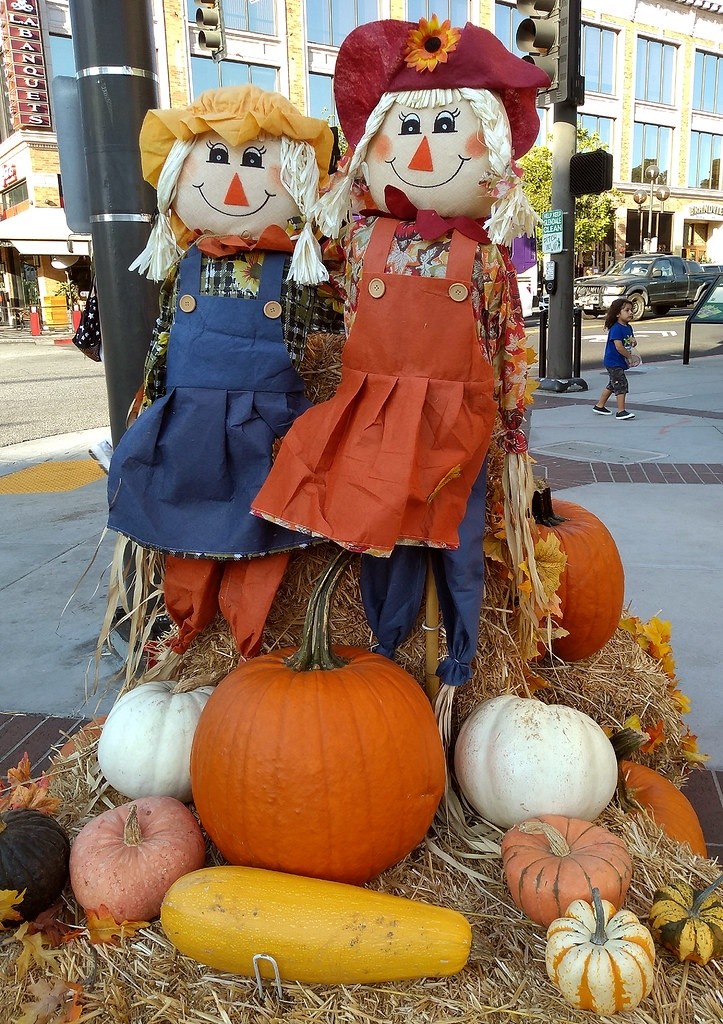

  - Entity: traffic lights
[569,152,606,194]
[516,0,560,95]
[194,0,221,52]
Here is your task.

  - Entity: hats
[334,18,552,161]
[140,83,333,191]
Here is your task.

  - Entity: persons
[250,17,553,685]
[592,299,636,421]
[104,84,344,663]
[655,264,668,276]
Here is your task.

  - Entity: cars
[539,261,723,310]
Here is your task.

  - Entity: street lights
[633,165,670,254]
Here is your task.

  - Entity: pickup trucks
[574,253,716,321]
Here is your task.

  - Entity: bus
[488,208,538,319]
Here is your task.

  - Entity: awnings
[9,239,90,256]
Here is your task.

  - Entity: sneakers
[592,405,612,415]
[615,409,635,419]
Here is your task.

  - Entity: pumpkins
[0,485,723,1014]
